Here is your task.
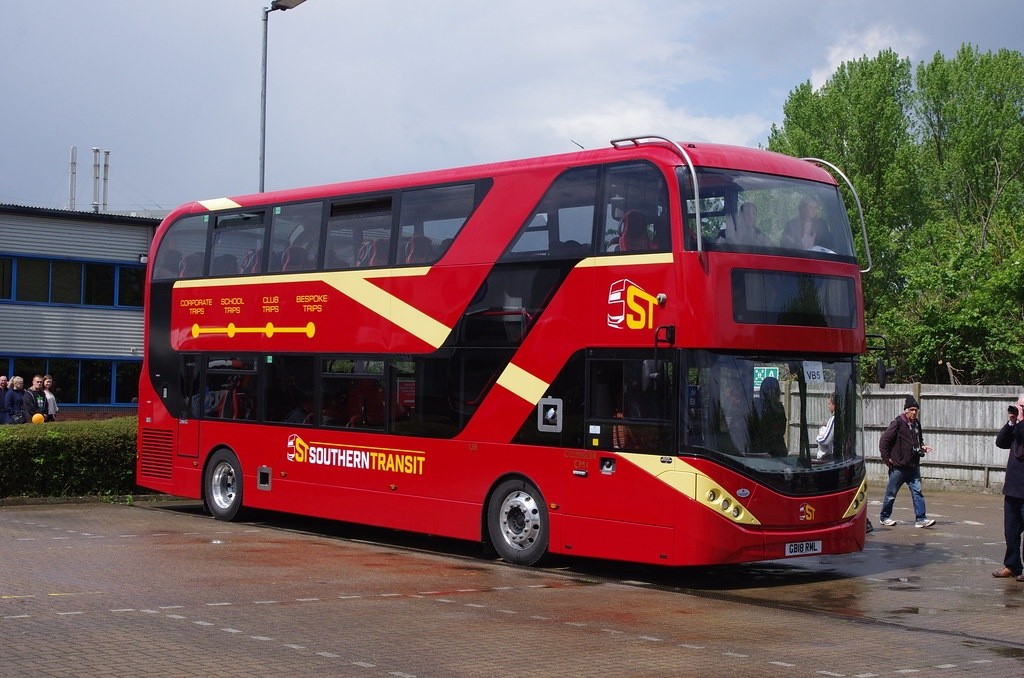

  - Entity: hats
[903,395,919,411]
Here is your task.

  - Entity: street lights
[258,0,306,193]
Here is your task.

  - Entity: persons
[992,395,1024,582]
[816,395,834,458]
[155,250,239,278]
[645,184,696,251]
[717,203,774,246]
[781,196,833,250]
[751,377,788,456]
[0,374,59,424]
[879,395,935,528]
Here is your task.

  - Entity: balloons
[32,413,44,425]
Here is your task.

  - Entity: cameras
[911,447,925,457]
[1008,405,1024,416]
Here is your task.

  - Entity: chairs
[615,210,674,255]
[156,238,457,279]
[193,379,413,436]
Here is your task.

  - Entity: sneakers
[879,517,897,526]
[915,519,936,528]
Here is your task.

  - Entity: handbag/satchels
[13,411,26,424]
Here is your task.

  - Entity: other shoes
[992,566,1024,580]
[866,523,874,533]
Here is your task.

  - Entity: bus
[134,135,891,568]
